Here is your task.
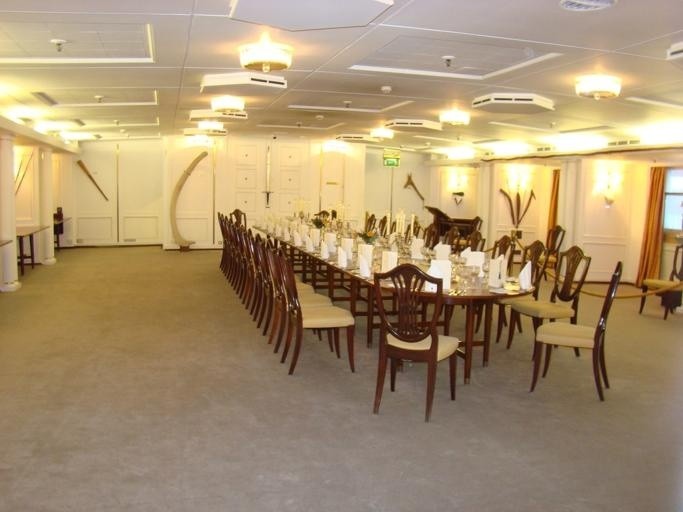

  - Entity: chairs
[639,246,683,319]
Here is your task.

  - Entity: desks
[0,217,72,275]
